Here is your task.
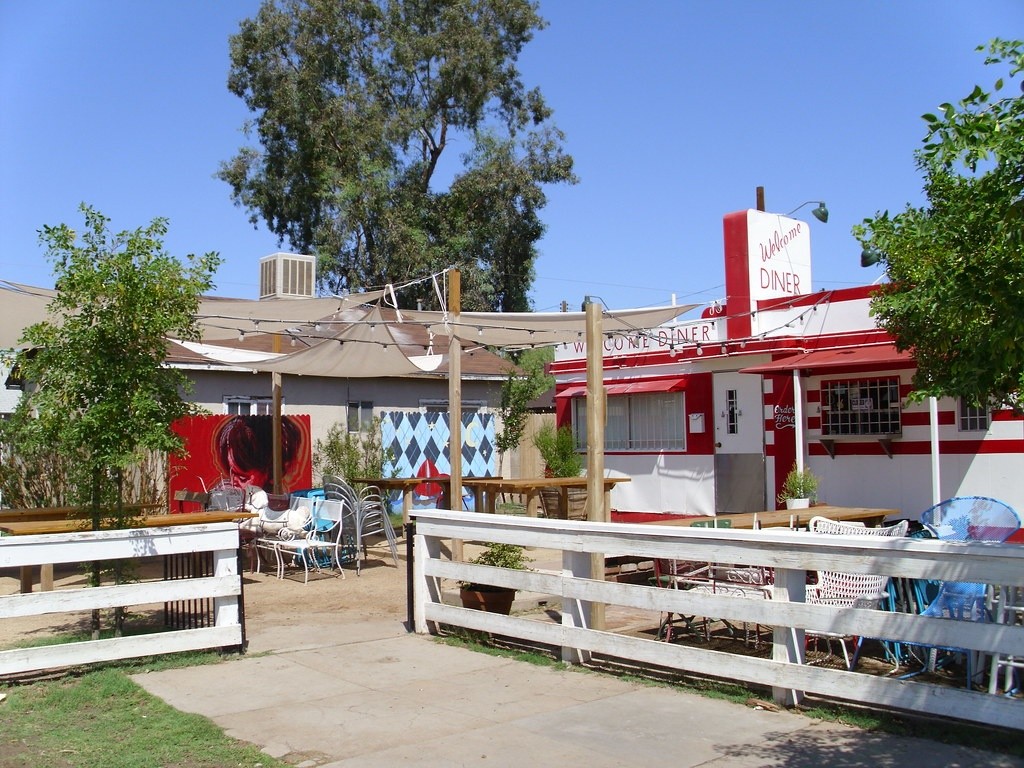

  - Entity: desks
[0,502,260,616]
[641,506,901,528]
[350,475,504,540]
[462,477,632,523]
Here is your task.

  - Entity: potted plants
[779,459,819,510]
[455,543,536,615]
[530,418,588,522]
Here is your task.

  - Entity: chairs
[758,516,909,677]
[277,496,346,586]
[886,496,1024,697]
[253,493,316,578]
[321,474,399,578]
